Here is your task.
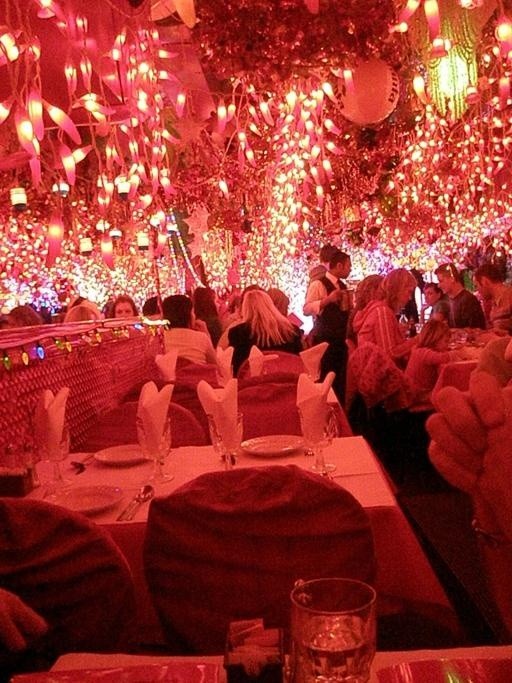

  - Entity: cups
[289,577,376,681]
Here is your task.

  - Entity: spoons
[127,482,155,521]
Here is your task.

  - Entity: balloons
[336,59,400,127]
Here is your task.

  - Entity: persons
[0,244,512,649]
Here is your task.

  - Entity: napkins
[35,385,69,448]
[196,378,239,449]
[296,369,334,440]
[248,345,278,376]
[153,351,178,380]
[136,381,174,448]
[301,341,329,370]
[216,344,234,379]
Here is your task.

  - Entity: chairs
[2,498,147,651]
[343,310,512,414]
[215,386,342,443]
[94,400,205,447]
[125,378,209,444]
[140,463,378,655]
[234,372,303,389]
[236,349,307,378]
[153,356,221,391]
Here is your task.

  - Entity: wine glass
[207,413,244,469]
[297,405,337,474]
[133,417,174,485]
[35,429,75,490]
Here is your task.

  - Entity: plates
[239,435,306,458]
[93,446,146,466]
[39,486,123,518]
